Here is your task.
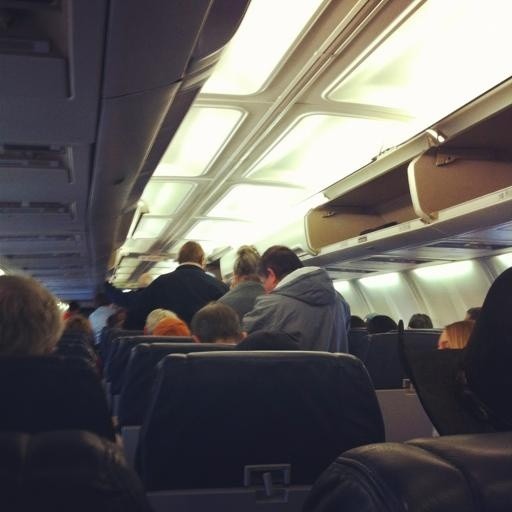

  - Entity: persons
[437,308,480,348]
[409,313,434,329]
[108,241,350,354]
[465,267,511,433]
[1,275,119,442]
[359,315,399,368]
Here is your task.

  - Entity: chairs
[55,322,512,512]
